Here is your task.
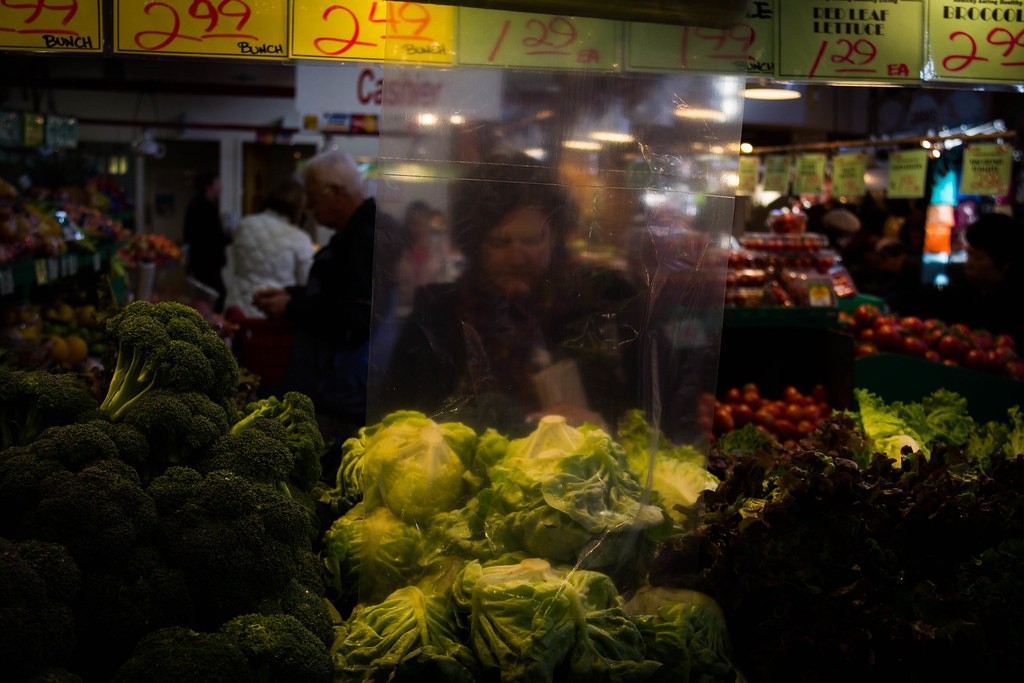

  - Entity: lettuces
[648,389,1023,683]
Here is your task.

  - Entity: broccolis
[0,299,359,683]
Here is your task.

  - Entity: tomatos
[692,303,1024,451]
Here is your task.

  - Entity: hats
[824,208,861,233]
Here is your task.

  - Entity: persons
[374,151,680,440]
[397,195,464,317]
[223,178,314,320]
[252,147,410,475]
[181,170,233,315]
[761,168,1024,358]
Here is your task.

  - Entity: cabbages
[327,406,738,683]
